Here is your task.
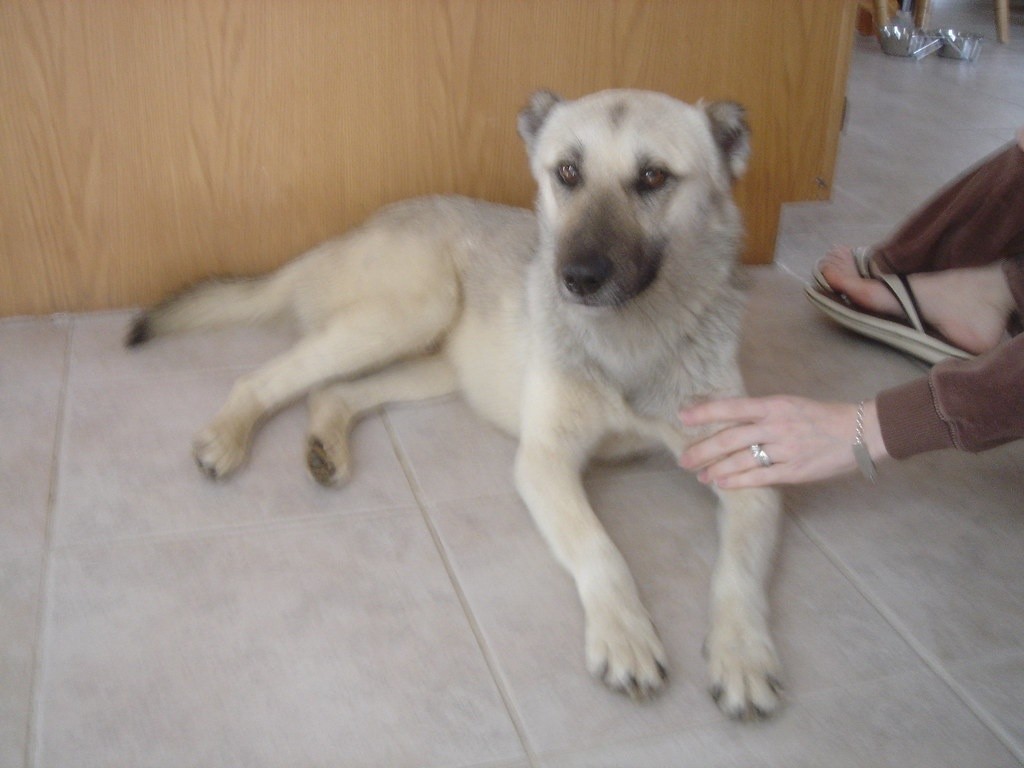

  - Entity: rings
[751,444,772,466]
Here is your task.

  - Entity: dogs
[123,88,785,721]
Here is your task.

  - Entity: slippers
[803,273,1017,366]
[814,245,870,294]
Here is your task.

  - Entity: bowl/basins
[879,25,933,58]
[939,27,984,60]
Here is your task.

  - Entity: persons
[677,119,1024,489]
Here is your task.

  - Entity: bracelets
[852,400,880,485]
